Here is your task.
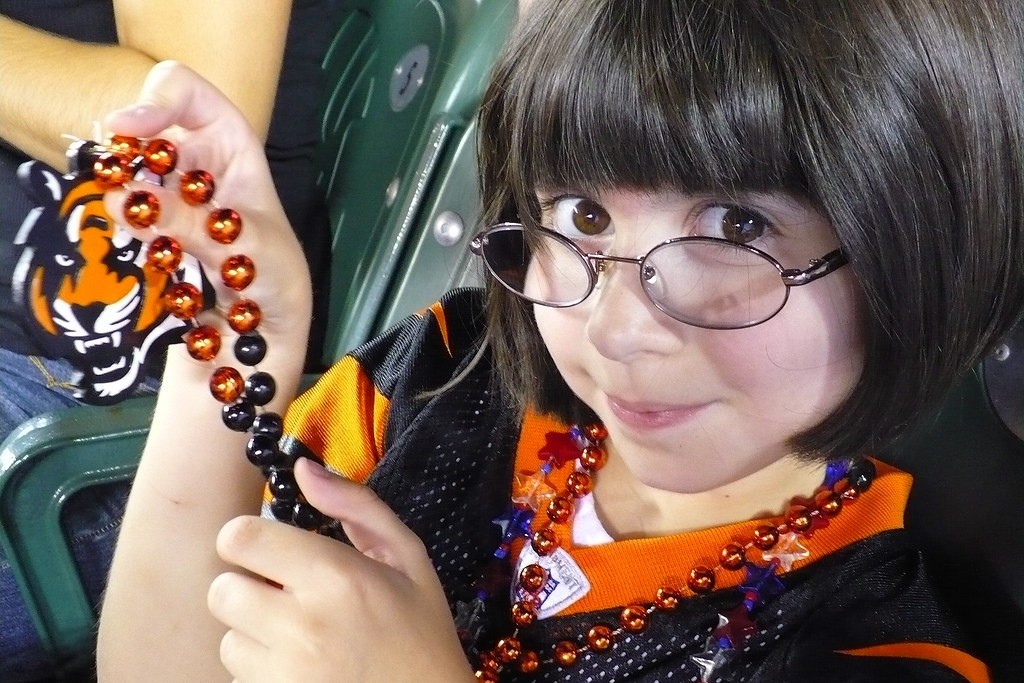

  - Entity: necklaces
[94,134,878,683]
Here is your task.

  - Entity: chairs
[0,0,520,683]
[882,362,1024,682]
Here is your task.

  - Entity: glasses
[469,211,849,331]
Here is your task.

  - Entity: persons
[94,0,1024,683]
[0,0,333,683]
